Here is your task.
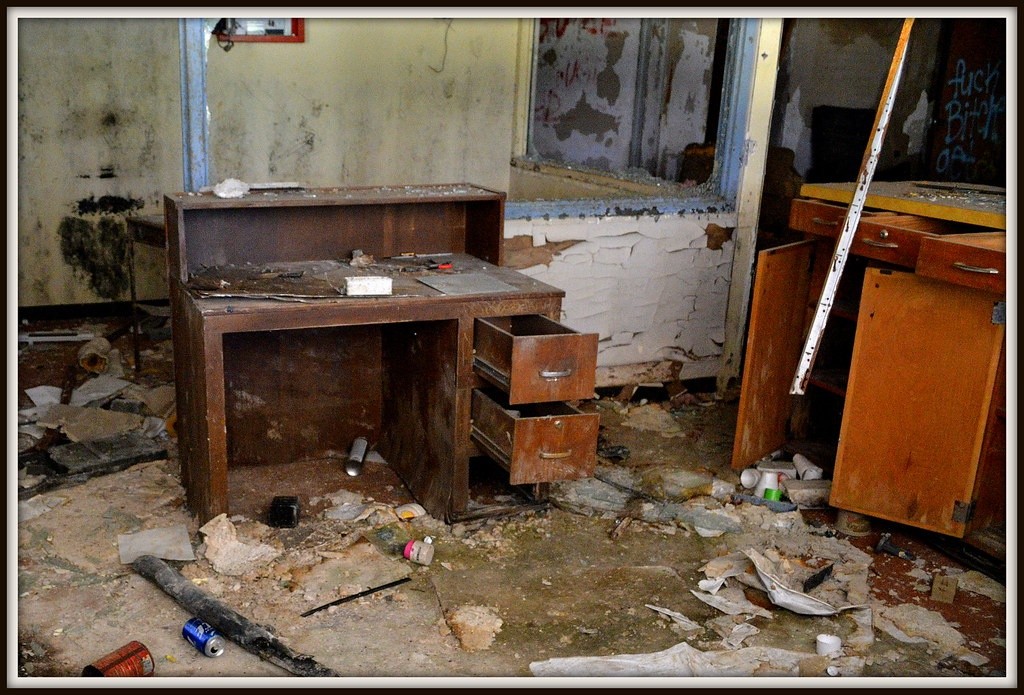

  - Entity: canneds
[182,616,226,657]
[344,436,368,476]
[82,640,155,677]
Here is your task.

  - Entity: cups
[754,471,778,499]
[741,469,761,489]
[792,453,820,480]
[816,634,841,655]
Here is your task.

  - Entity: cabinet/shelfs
[162,183,600,542]
[731,182,1007,560]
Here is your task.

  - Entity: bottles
[345,436,368,476]
